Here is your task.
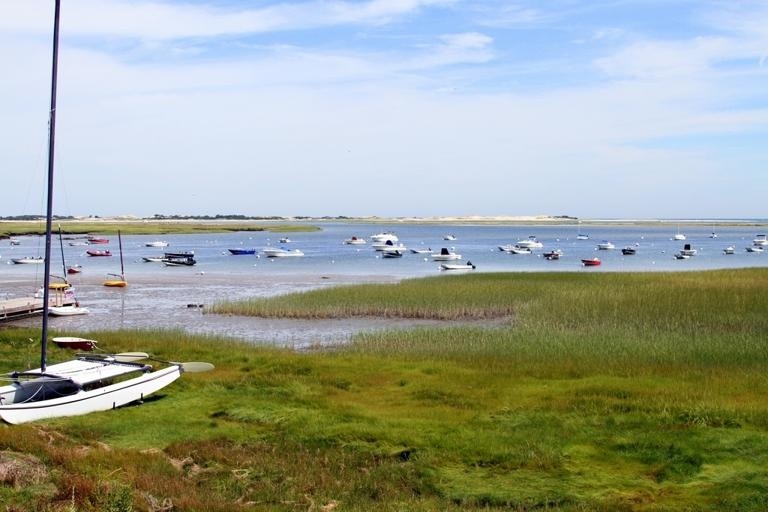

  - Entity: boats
[142,235,197,274]
[724,234,768,255]
[577,233,636,266]
[497,234,560,260]
[674,234,697,259]
[52,337,98,350]
[709,232,717,238]
[0,233,127,321]
[0,0,215,425]
[345,230,476,269]
[228,237,305,257]
